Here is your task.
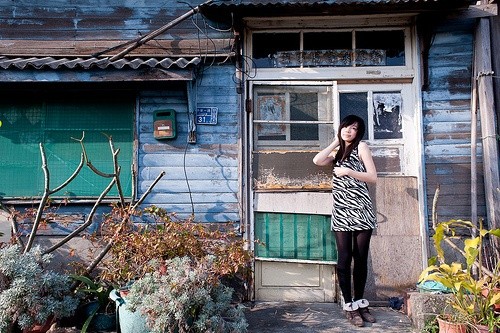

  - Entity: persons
[313,114,377,327]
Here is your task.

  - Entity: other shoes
[343,302,365,327]
[355,298,375,323]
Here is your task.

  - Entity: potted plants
[0,243,80,333]
[109,254,249,333]
[416,216,500,333]
